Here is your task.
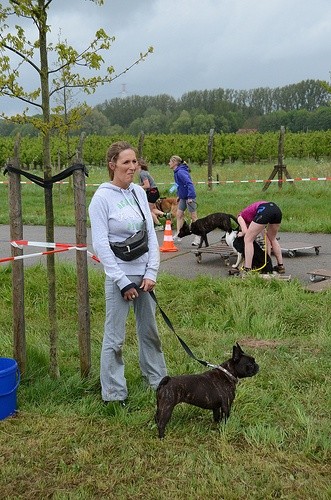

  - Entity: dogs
[178,211,242,248]
[156,341,259,434]
[223,227,273,278]
[146,198,178,224]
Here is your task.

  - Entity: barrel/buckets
[0,357,20,422]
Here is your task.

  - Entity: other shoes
[272,265,284,273]
[153,224,163,231]
[164,213,168,220]
[171,236,181,241]
[191,240,200,246]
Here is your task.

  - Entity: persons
[135,158,172,232]
[87,140,168,408]
[168,155,201,245]
[236,201,286,277]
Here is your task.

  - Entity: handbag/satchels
[146,187,160,204]
[109,220,148,261]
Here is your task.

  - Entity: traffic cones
[160,220,178,252]
